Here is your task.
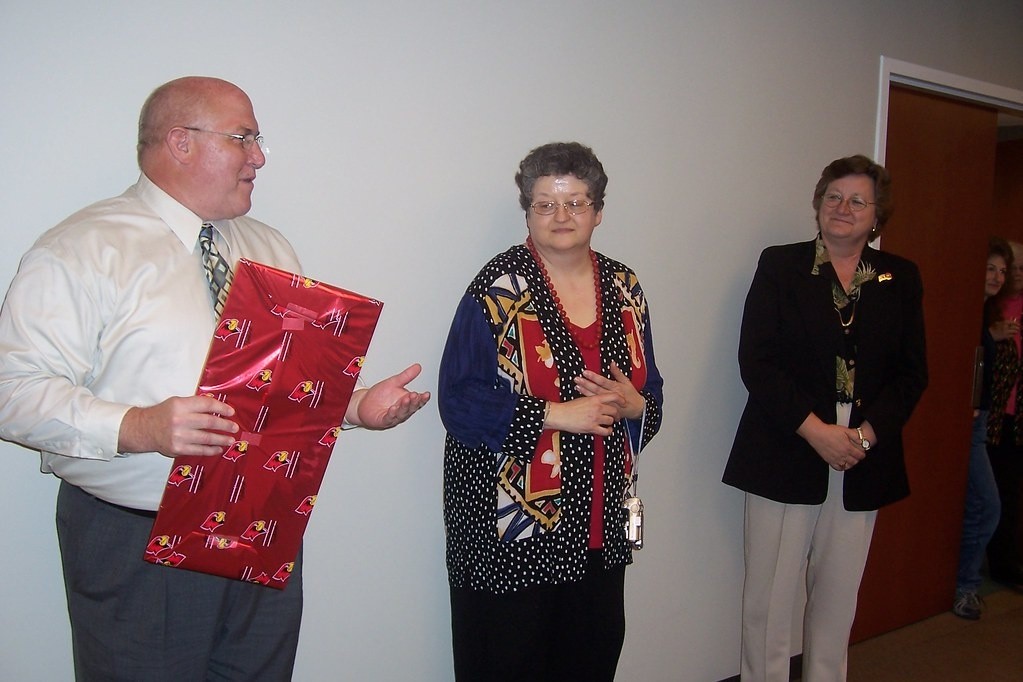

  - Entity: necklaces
[527,234,603,351]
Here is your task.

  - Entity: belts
[80,489,158,519]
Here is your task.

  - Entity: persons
[0,76,431,682]
[721,154,929,682]
[438,142,664,682]
[952,238,1023,620]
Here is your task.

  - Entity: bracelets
[544,401,552,423]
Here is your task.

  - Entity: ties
[199,223,235,323]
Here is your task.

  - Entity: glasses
[821,194,875,211]
[530,200,594,216]
[184,127,263,151]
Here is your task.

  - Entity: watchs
[857,427,870,452]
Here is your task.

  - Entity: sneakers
[951,587,987,619]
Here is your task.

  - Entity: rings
[838,463,848,469]
